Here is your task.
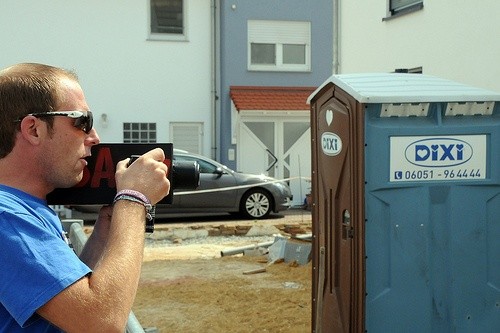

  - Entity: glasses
[13,110,93,134]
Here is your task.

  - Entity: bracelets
[114,189,151,205]
[113,195,153,221]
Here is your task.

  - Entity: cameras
[130,155,156,233]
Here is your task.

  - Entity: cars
[64,149,293,224]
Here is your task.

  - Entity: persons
[0,62,172,333]
